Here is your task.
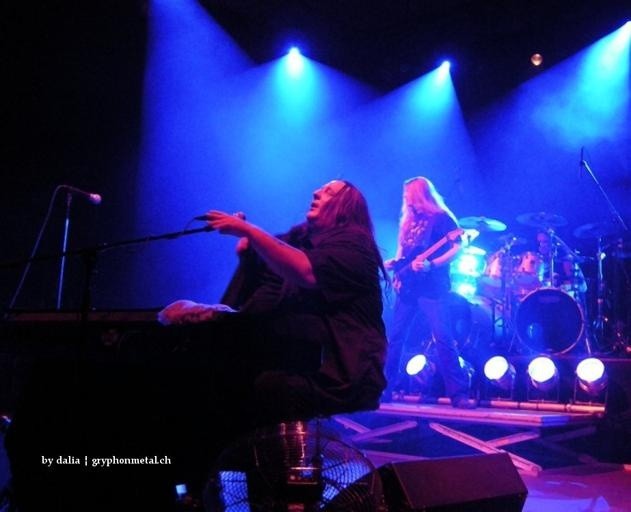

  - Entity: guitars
[391,226,466,298]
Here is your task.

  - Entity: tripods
[487,225,630,358]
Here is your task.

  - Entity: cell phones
[174,482,189,503]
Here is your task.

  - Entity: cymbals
[573,221,624,241]
[456,216,507,233]
[515,209,571,229]
[554,254,587,293]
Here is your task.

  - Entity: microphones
[63,184,102,205]
[195,211,246,221]
[579,146,584,178]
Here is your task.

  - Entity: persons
[152,177,630,511]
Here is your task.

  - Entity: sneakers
[456,399,475,408]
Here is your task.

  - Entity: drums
[481,251,513,288]
[514,252,546,286]
[513,287,585,357]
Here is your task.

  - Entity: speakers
[322,451,528,512]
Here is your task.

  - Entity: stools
[259,383,387,468]
